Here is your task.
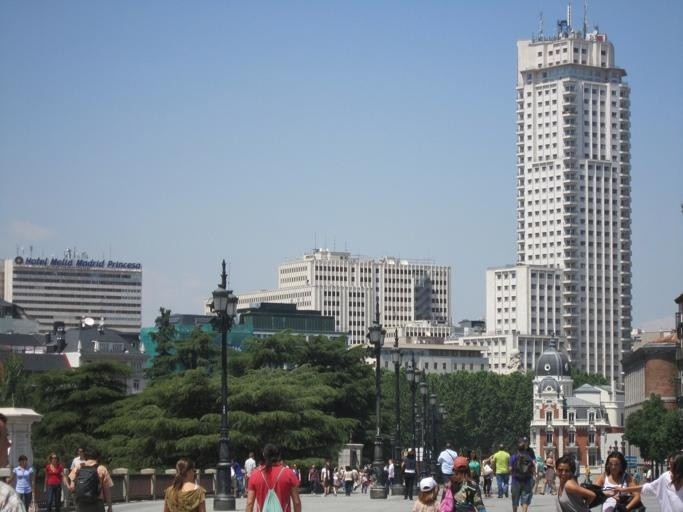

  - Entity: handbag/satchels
[438,480,454,511]
[614,493,646,512]
[580,482,621,508]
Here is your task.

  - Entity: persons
[437,443,511,499]
[442,456,486,512]
[402,450,420,499]
[164,457,206,512]
[597,452,640,512]
[383,459,394,495]
[0,445,114,512]
[246,443,301,512]
[509,437,557,512]
[583,464,591,484]
[413,477,442,512]
[614,451,683,512]
[634,469,640,486]
[555,456,596,512]
[646,466,652,483]
[231,451,257,498]
[285,463,373,496]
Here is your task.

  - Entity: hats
[454,456,469,471]
[419,477,437,491]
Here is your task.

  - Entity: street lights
[209,259,238,512]
[367,304,447,499]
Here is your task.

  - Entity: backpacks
[75,463,101,504]
[513,454,533,481]
[256,466,289,512]
